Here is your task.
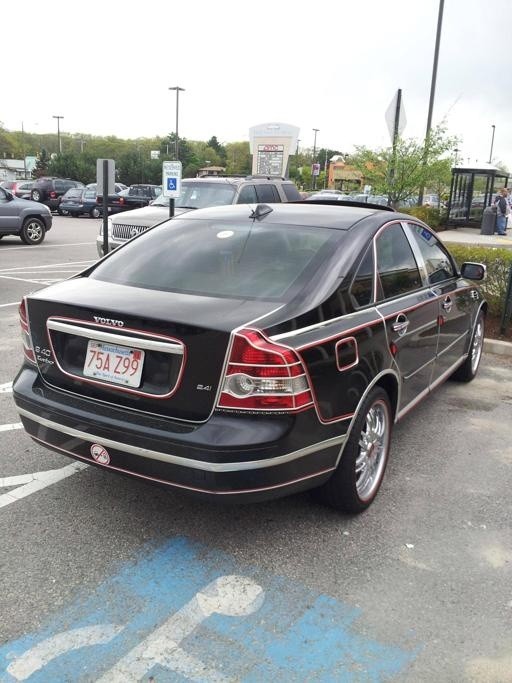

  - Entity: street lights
[309,128,321,193]
[52,115,65,154]
[169,85,185,160]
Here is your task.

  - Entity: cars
[300,189,512,218]
[10,201,491,514]
[0,176,164,246]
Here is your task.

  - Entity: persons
[493,188,510,235]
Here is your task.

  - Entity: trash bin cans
[481,207,497,235]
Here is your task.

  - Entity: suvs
[96,173,307,259]
